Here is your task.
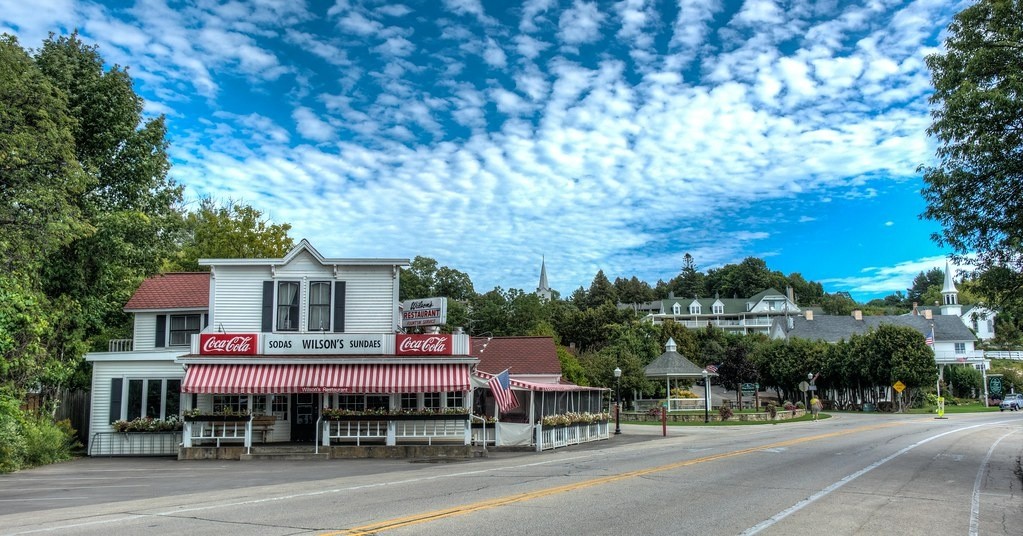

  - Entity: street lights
[701,369,709,423]
[807,373,814,413]
[614,366,622,434]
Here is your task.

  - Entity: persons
[810,395,822,420]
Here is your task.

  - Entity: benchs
[205,416,276,442]
[330,421,387,443]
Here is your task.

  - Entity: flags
[922,331,933,345]
[706,364,721,373]
[488,367,520,414]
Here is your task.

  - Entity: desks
[727,400,753,409]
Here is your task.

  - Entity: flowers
[321,405,471,420]
[181,407,247,417]
[112,414,182,433]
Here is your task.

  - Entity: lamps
[473,344,486,354]
[471,331,494,342]
[473,339,489,349]
[218,322,226,334]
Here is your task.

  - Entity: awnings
[182,364,471,395]
[475,371,611,423]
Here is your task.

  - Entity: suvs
[999,394,1023,411]
